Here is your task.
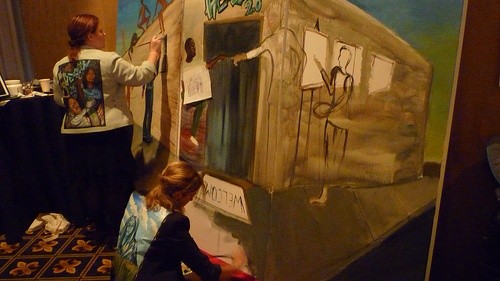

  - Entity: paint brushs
[29,76,36,93]
[210,252,234,261]
[135,34,167,48]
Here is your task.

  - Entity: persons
[115,156,246,281]
[52,14,164,238]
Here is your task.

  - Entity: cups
[39,79,50,92]
